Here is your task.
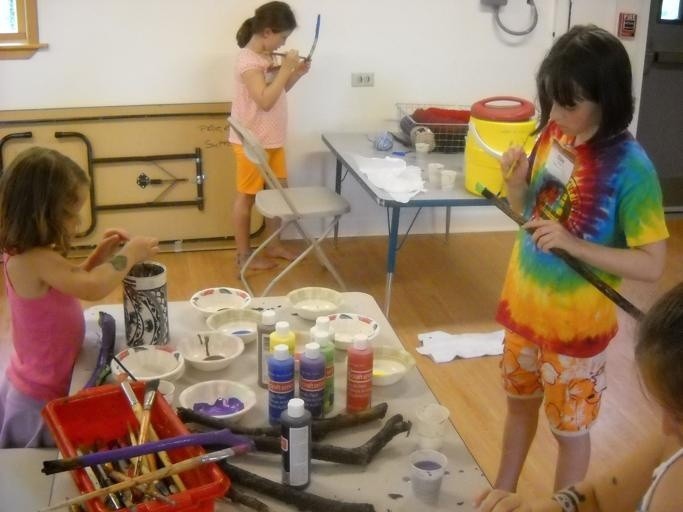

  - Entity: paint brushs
[36,353,256,512]
[475,182,647,321]
[497,134,530,197]
[264,52,306,59]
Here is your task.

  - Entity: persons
[474,281,683,512]
[229,1,311,271]
[493,24,669,494]
[0,147,160,449]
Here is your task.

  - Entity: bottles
[271,322,295,359]
[263,310,276,351]
[278,399,312,489]
[345,334,374,414]
[310,317,335,412]
[266,343,295,424]
[300,341,326,418]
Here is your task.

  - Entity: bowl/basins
[157,381,175,408]
[344,346,412,388]
[177,332,245,372]
[324,314,379,351]
[288,286,343,320]
[189,287,251,318]
[179,379,257,423]
[112,345,186,385]
[207,309,263,345]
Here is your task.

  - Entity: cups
[408,401,450,508]
[416,142,457,194]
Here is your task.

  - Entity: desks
[0,100,266,249]
[321,129,511,321]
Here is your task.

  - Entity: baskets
[394,103,470,153]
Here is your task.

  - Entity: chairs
[223,114,352,298]
[0,447,58,512]
[46,291,495,512]
